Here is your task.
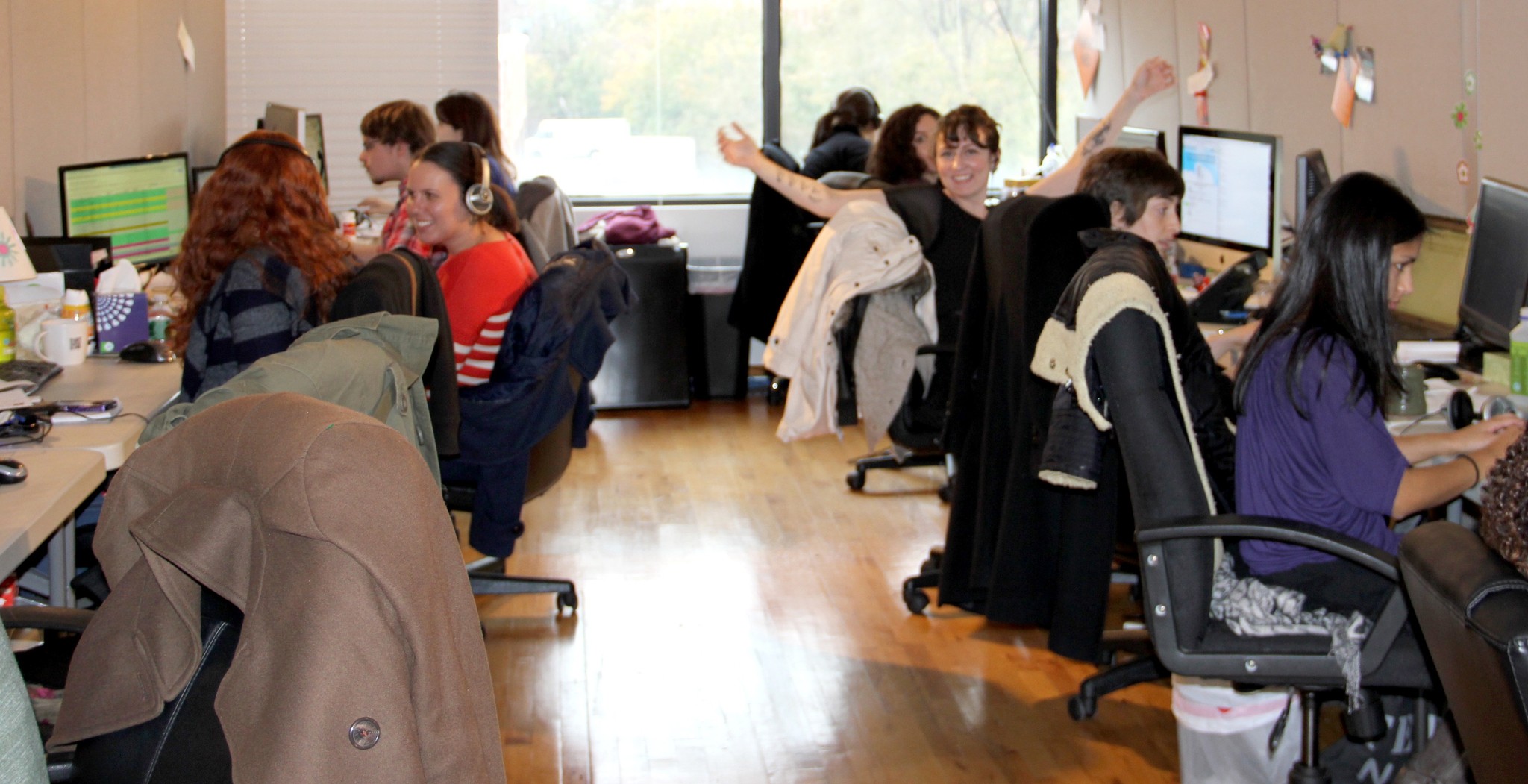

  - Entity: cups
[1385,363,1427,416]
[34,317,87,366]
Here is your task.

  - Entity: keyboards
[0,358,62,397]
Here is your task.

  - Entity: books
[50,406,123,424]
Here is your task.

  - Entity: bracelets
[1456,453,1479,489]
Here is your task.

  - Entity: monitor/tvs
[1177,125,1281,281]
[1293,148,1330,240]
[1458,176,1528,350]
[257,102,329,195]
[1073,116,1167,158]
[20,151,219,320]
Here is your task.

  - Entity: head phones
[1438,389,1517,430]
[464,142,498,214]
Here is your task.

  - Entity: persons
[12,128,365,604]
[437,90,518,197]
[336,98,449,271]
[717,55,1174,424]
[1480,424,1528,578]
[405,139,538,387]
[1076,147,1262,516]
[1231,172,1528,638]
[803,87,880,180]
[866,102,941,188]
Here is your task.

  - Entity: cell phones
[49,399,117,411]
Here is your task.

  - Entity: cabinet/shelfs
[584,241,690,409]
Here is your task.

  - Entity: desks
[0,354,182,607]
[0,449,106,607]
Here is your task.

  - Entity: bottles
[0,285,16,365]
[339,210,357,242]
[1041,142,1060,177]
[62,288,97,357]
[1508,307,1528,420]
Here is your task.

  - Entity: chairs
[727,140,1527,784]
[0,175,639,784]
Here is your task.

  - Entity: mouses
[0,457,28,484]
